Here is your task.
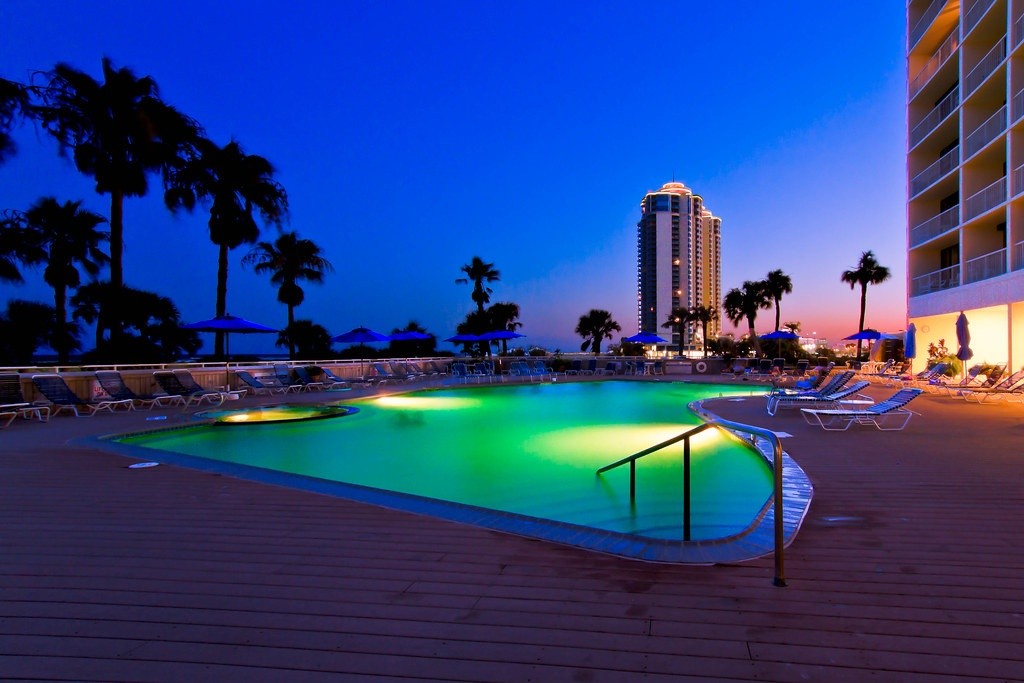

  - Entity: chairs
[0,346,1024,435]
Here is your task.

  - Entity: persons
[774,370,827,389]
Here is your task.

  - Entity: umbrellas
[478,330,528,383]
[841,329,899,362]
[175,317,281,400]
[387,332,435,374]
[327,328,389,387]
[759,331,802,368]
[444,334,482,358]
[623,333,668,359]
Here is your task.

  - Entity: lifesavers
[696,362,707,373]
